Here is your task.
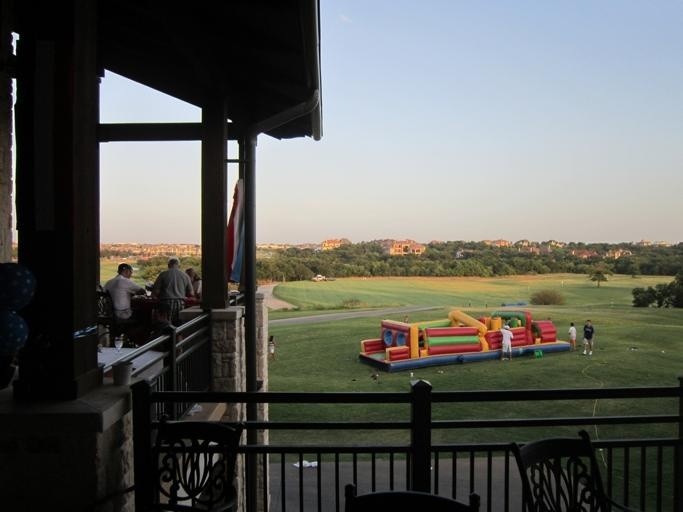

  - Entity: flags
[227,179,246,284]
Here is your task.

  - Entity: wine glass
[113,336,123,356]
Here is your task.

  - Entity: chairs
[98,285,188,347]
[344,482,479,512]
[153,410,245,511]
[508,428,612,512]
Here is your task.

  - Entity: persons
[582,320,594,356]
[268,335,276,361]
[404,315,409,323]
[104,255,202,327]
[499,325,514,361]
[568,322,576,352]
